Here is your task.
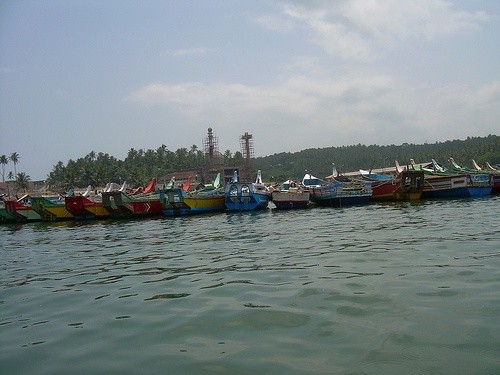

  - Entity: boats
[301,163,424,206]
[159,171,245,215]
[0,185,101,221]
[394,157,500,199]
[272,180,310,208]
[224,170,275,212]
[60,177,163,221]
